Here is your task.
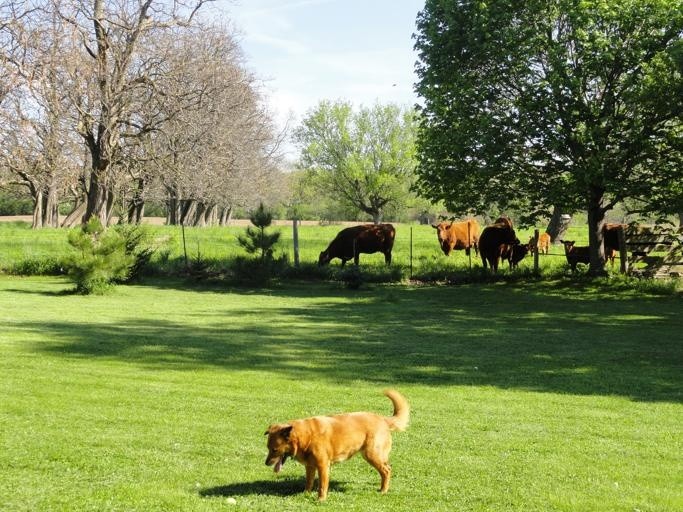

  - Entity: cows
[317,222,396,269]
[430,219,481,256]
[481,216,552,273]
[559,222,669,273]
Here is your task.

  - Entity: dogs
[263,387,412,502]
